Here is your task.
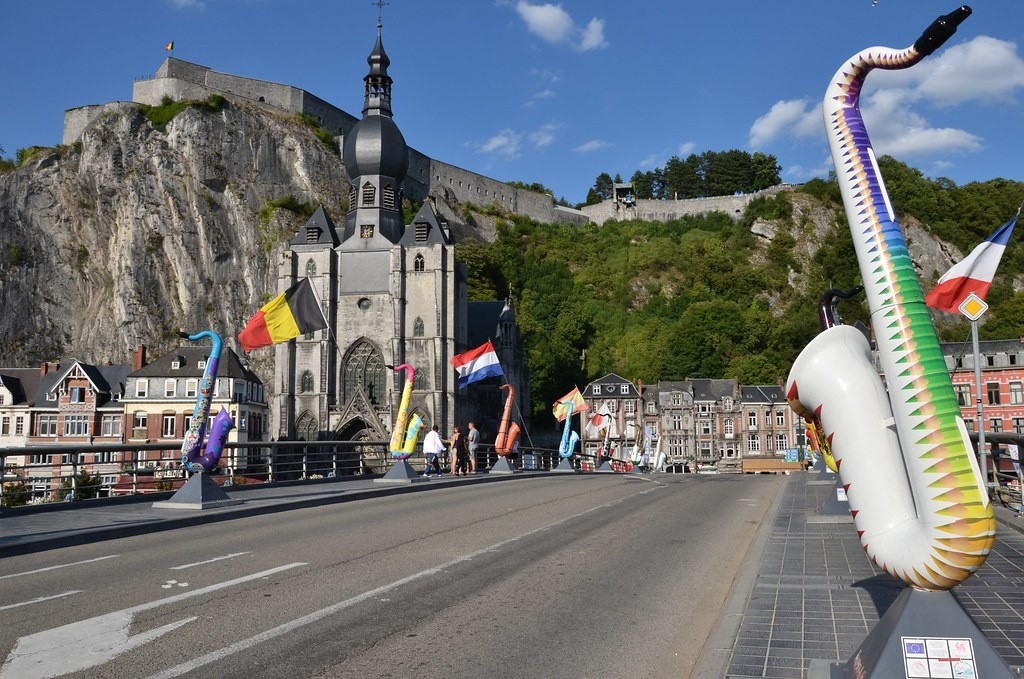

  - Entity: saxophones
[181,331,234,476]
[492,385,521,455]
[778,6,995,588]
[628,423,646,464]
[596,413,617,461]
[556,400,580,459]
[805,416,839,475]
[652,432,668,473]
[384,363,425,460]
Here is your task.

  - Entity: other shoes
[438,473,444,477]
[450,471,476,476]
[423,473,432,478]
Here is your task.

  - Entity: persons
[422,425,446,477]
[447,421,480,476]
[509,440,521,470]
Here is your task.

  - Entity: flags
[585,402,616,434]
[552,386,590,422]
[449,341,505,390]
[237,274,331,352]
[923,211,1021,315]
